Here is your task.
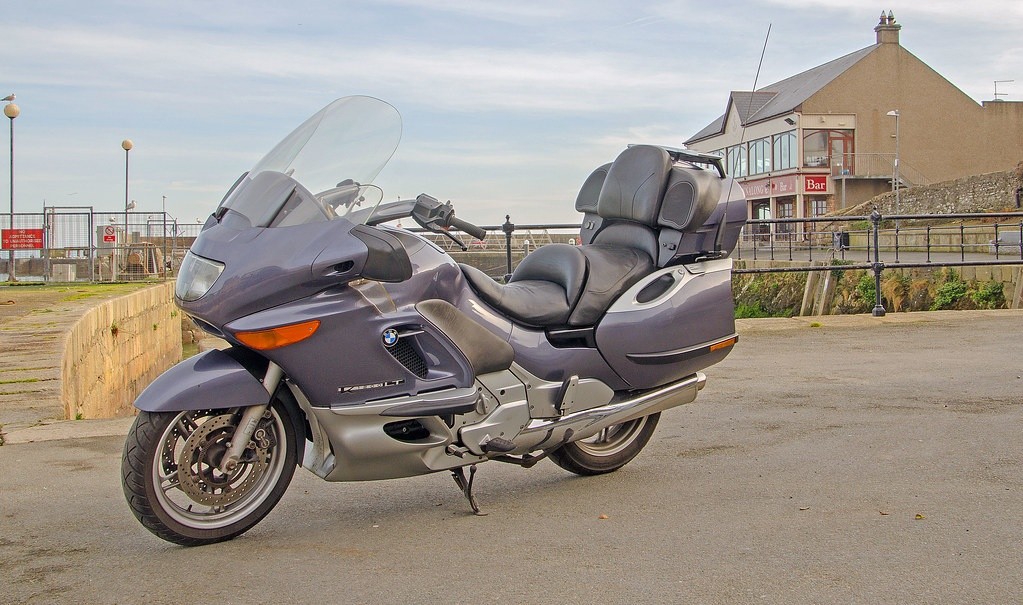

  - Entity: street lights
[4,104,19,282]
[887,111,898,228]
[122,140,133,266]
[162,196,167,211]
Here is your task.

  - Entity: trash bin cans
[53,264,77,283]
[837,232,850,250]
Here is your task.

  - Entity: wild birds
[0,93,16,104]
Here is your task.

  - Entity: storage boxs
[575,159,747,266]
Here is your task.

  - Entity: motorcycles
[122,96,746,547]
